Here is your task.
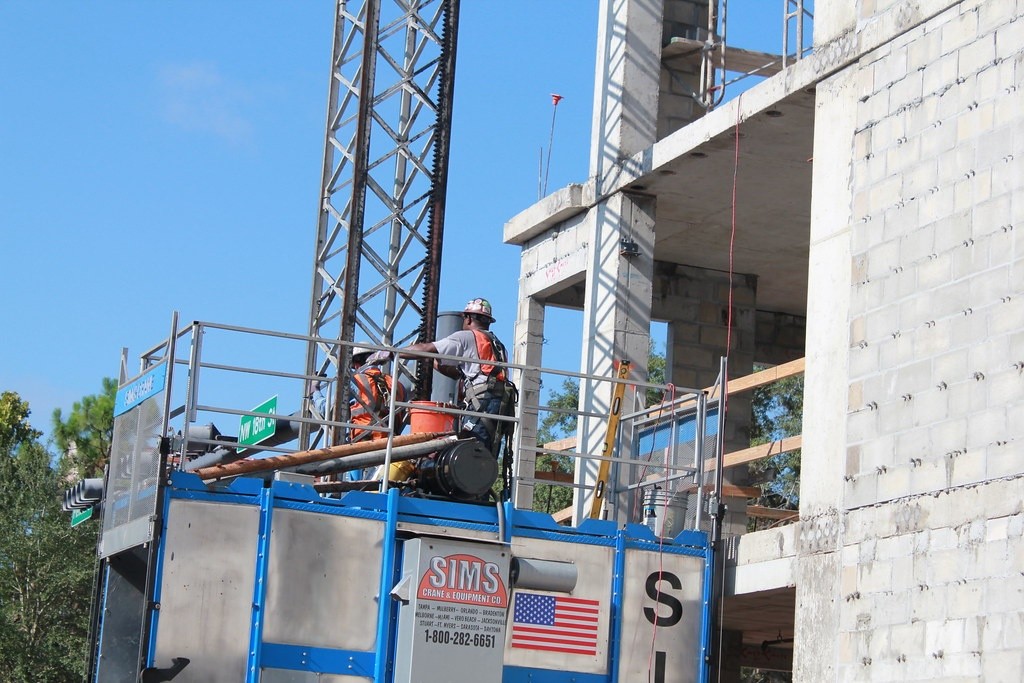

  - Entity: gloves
[365,347,393,368]
[308,371,327,397]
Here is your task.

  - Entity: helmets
[458,298,499,321]
[348,341,378,357]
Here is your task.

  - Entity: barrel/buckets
[410,401,459,434]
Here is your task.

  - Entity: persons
[366,298,509,453]
[307,340,409,451]
